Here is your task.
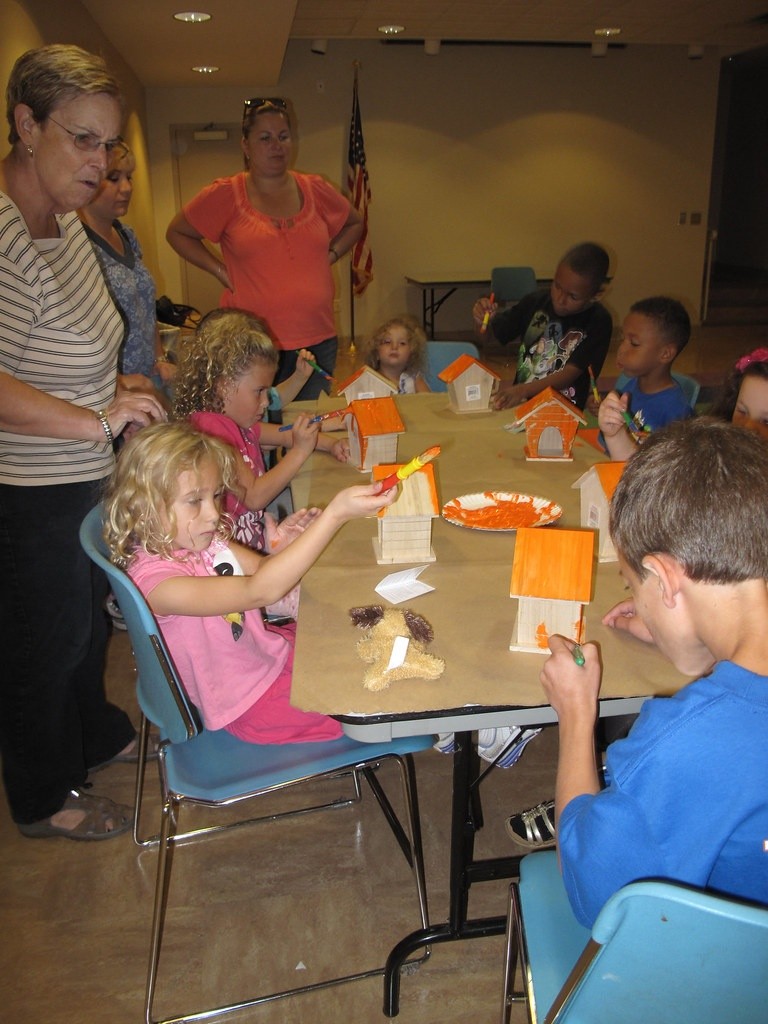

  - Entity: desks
[404,264,613,342]
[281,390,712,1016]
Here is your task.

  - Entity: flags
[345,84,374,296]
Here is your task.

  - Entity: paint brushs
[481,293,495,333]
[587,366,601,402]
[622,412,640,435]
[573,643,585,666]
[372,445,441,495]
[279,407,351,431]
[294,349,334,382]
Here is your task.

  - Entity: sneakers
[478,727,543,769]
[433,733,454,754]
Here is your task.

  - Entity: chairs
[78,495,431,1024]
[501,852,768,1024]
[401,341,477,395]
[613,368,701,410]
[489,266,536,322]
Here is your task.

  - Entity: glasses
[47,114,128,163]
[242,98,288,124]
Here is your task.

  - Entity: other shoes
[106,598,127,630]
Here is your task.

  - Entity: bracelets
[328,248,339,260]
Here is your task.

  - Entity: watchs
[95,410,115,445]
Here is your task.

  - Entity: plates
[442,492,564,532]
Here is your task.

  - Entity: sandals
[18,790,135,840]
[88,734,160,773]
[505,802,556,849]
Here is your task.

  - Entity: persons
[472,244,613,413]
[165,98,365,401]
[0,43,168,842]
[587,296,692,456]
[505,347,768,850]
[483,422,768,927]
[75,129,547,768]
